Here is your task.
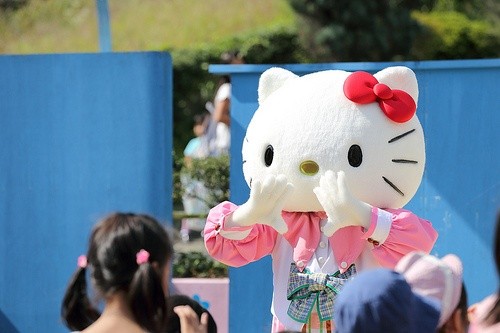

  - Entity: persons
[331,269,441,333]
[166,295,217,333]
[395,252,470,333]
[60,212,208,333]
[467,210,499,333]
[180,114,212,240]
[206,83,231,203]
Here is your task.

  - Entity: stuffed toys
[202,65,439,333]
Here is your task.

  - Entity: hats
[333,268,440,332]
[396,251,463,330]
